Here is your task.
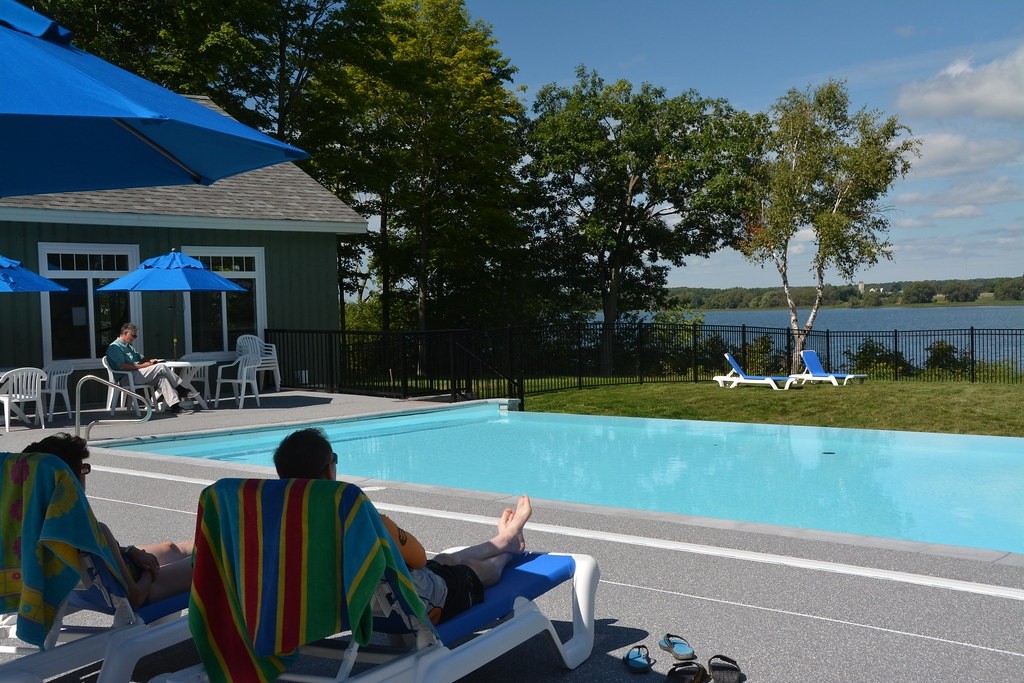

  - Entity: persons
[274,428,532,635]
[107,324,200,415]
[24,431,195,608]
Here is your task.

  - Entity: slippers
[708,655,741,683]
[623,645,652,675]
[659,633,695,660]
[662,662,706,683]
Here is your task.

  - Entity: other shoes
[179,389,200,399]
[170,407,195,415]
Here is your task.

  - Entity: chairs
[0,362,74,431]
[0,453,602,683]
[714,350,867,391]
[102,335,282,416]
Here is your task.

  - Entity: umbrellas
[0,0,312,197]
[98,248,248,372]
[0,256,69,292]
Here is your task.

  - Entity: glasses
[129,332,137,338]
[72,463,91,475]
[326,453,338,466]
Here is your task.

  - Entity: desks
[145,360,217,410]
